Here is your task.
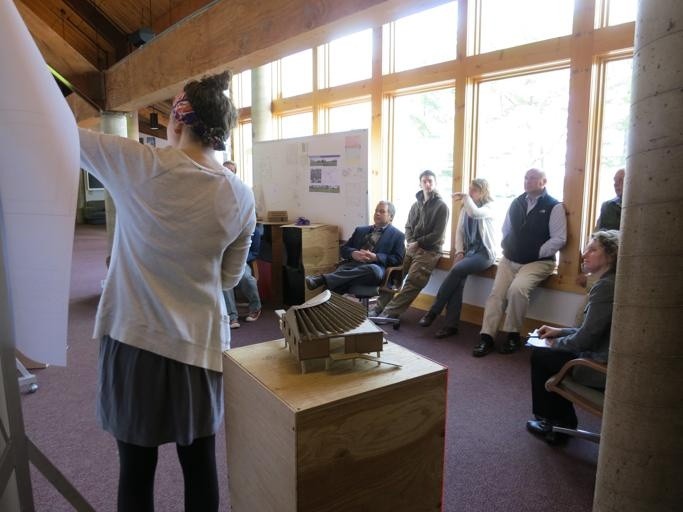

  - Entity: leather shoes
[419,309,436,327]
[306,274,324,290]
[434,326,457,339]
[502,335,521,354]
[526,420,572,447]
[473,338,494,356]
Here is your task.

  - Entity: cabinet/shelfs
[221,332,449,512]
[280,222,336,303]
[256,220,281,309]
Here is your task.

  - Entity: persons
[419,180,501,338]
[369,171,450,325]
[473,167,565,357]
[77,72,257,512]
[594,168,624,230]
[304,200,406,294]
[222,225,263,329]
[524,231,618,446]
[222,161,237,176]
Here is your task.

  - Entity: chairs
[349,248,407,331]
[542,358,607,448]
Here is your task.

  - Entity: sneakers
[375,312,399,325]
[230,319,240,328]
[245,310,262,321]
